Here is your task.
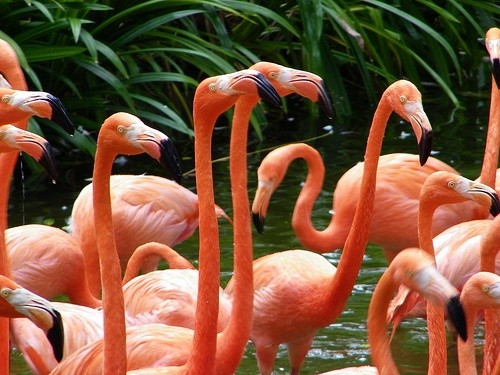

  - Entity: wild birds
[0,25,500,375]
